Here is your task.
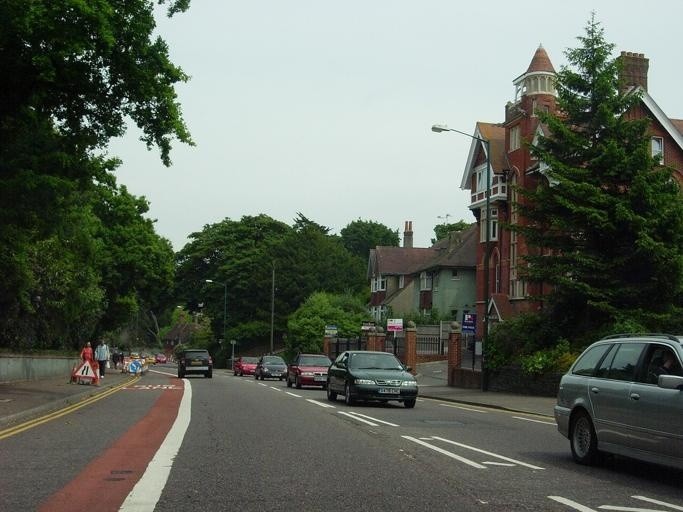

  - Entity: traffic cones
[89,363,100,386]
[65,362,78,385]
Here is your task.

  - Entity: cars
[253,355,287,383]
[325,351,419,408]
[234,356,258,378]
[286,353,334,391]
[119,350,166,366]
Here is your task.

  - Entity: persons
[650,351,677,384]
[93,337,110,379]
[80,340,93,362]
[112,350,120,370]
[116,353,122,368]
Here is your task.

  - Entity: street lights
[177,305,198,328]
[431,120,491,397]
[203,279,226,357]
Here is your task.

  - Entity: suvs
[176,349,212,379]
[554,330,682,473]
